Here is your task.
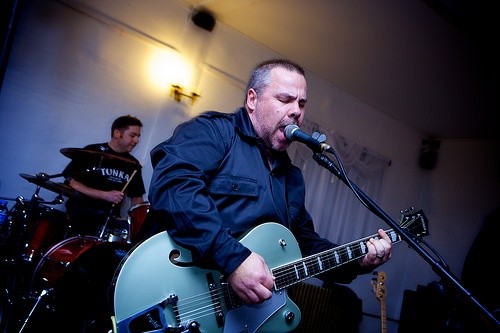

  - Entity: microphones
[283,124,335,155]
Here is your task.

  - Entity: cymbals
[59,147,136,167]
[19,172,80,200]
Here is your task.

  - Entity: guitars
[110,203,430,333]
[371,270,388,333]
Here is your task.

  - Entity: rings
[376,254,385,259]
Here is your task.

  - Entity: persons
[137,58,395,306]
[63,114,146,226]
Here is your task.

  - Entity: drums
[6,201,70,265]
[30,234,125,328]
[126,201,151,243]
